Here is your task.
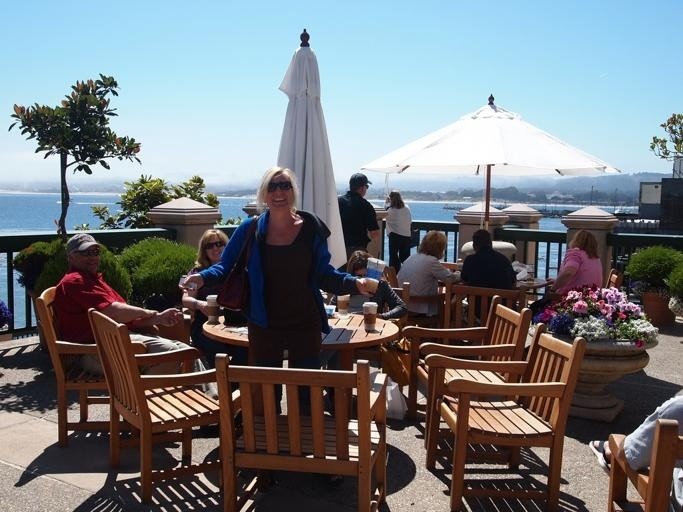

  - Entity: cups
[363,257,386,293]
[336,294,350,316]
[525,264,535,284]
[324,305,336,318]
[363,302,378,331]
[455,259,463,271]
[206,295,220,324]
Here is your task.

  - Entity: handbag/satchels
[216,264,249,313]
[380,337,412,387]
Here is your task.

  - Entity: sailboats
[440,187,638,224]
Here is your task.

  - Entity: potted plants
[625,245,683,328]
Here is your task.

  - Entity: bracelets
[151,312,159,324]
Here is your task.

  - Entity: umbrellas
[362,94,622,228]
[277,28,347,269]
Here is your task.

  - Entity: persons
[181,228,246,368]
[179,166,379,494]
[588,389,683,512]
[396,230,462,321]
[335,172,379,272]
[54,232,242,436]
[461,228,517,328]
[383,192,412,275]
[529,229,603,325]
[327,251,407,416]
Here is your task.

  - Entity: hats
[349,173,373,186]
[66,233,101,257]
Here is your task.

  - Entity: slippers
[589,440,611,478]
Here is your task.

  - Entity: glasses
[204,241,224,249]
[78,249,101,257]
[267,181,292,192]
